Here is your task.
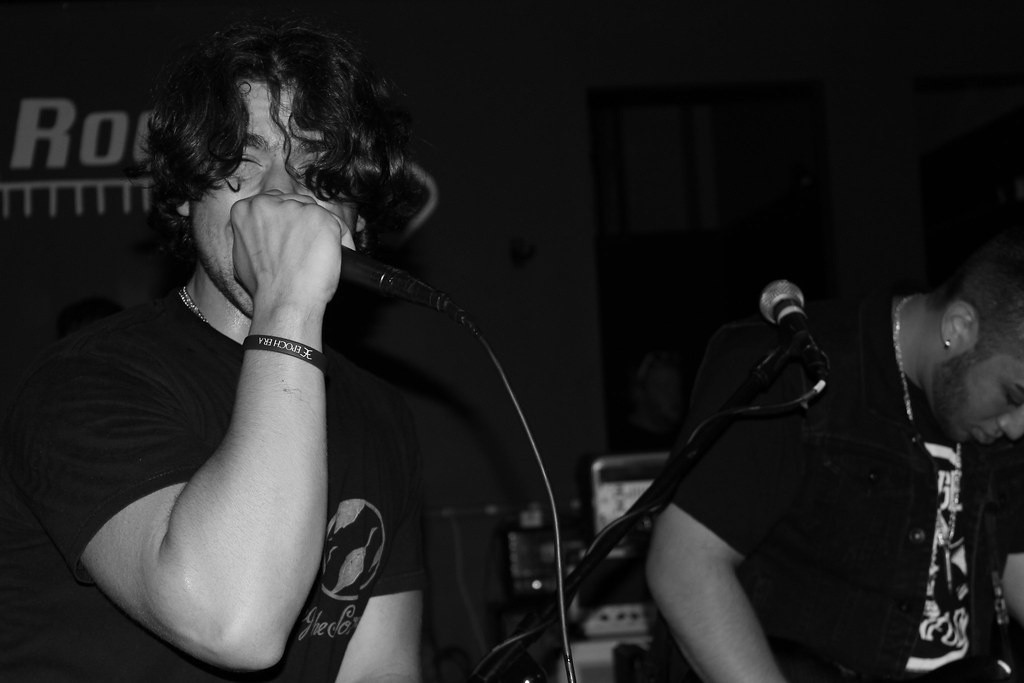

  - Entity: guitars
[677,633,1015,683]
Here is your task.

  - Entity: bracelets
[242,334,327,375]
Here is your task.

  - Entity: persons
[645,233,1024,683]
[0,21,529,683]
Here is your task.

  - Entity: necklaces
[177,287,209,323]
[893,294,962,546]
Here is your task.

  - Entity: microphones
[338,244,465,321]
[760,279,829,381]
